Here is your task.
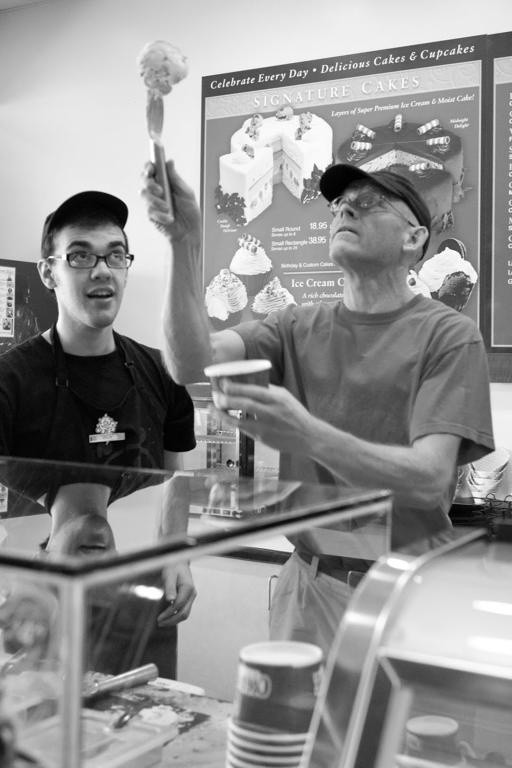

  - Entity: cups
[204,360,271,407]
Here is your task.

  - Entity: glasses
[327,192,416,227]
[48,251,133,268]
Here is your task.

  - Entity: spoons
[145,96,180,223]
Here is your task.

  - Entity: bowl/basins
[221,642,327,768]
[465,444,509,504]
[396,716,468,768]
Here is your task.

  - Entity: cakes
[250,273,298,320]
[227,232,276,296]
[204,266,249,330]
[405,238,479,312]
[335,111,465,234]
[213,105,333,226]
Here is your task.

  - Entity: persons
[140,157,495,666]
[0,189,199,681]
[0,458,174,562]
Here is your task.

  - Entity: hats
[319,163,431,260]
[41,190,128,245]
